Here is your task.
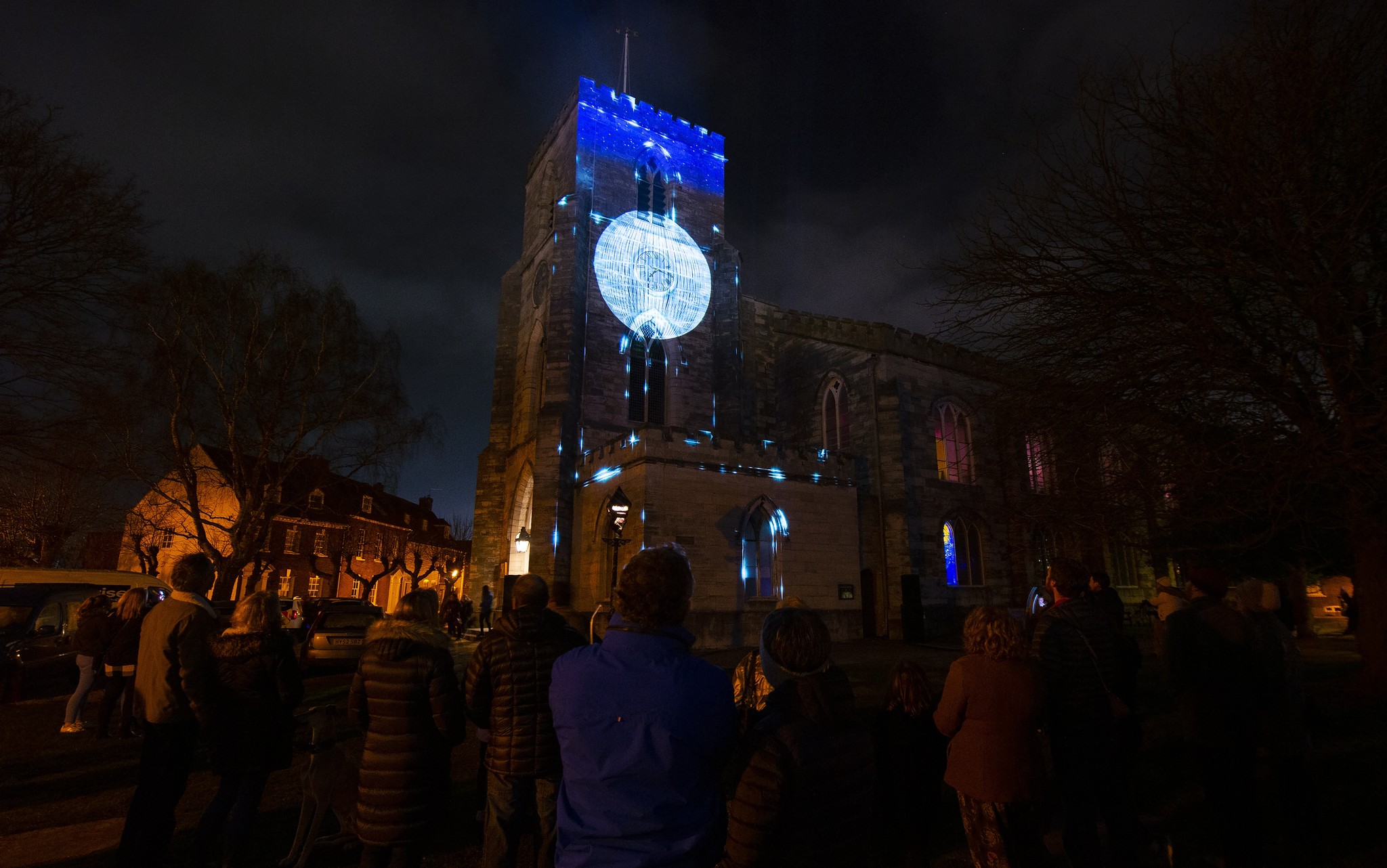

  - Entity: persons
[879,557,1299,868]
[59,588,153,740]
[548,548,740,868]
[439,575,589,868]
[715,608,883,868]
[439,585,491,639]
[117,553,307,868]
[347,589,467,868]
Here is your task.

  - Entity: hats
[1188,567,1228,598]
[1226,578,1280,613]
[1157,576,1171,587]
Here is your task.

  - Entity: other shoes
[476,633,484,637]
[97,729,113,739]
[456,637,461,640]
[121,731,141,738]
[60,720,87,733]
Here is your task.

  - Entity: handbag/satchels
[1107,695,1130,719]
